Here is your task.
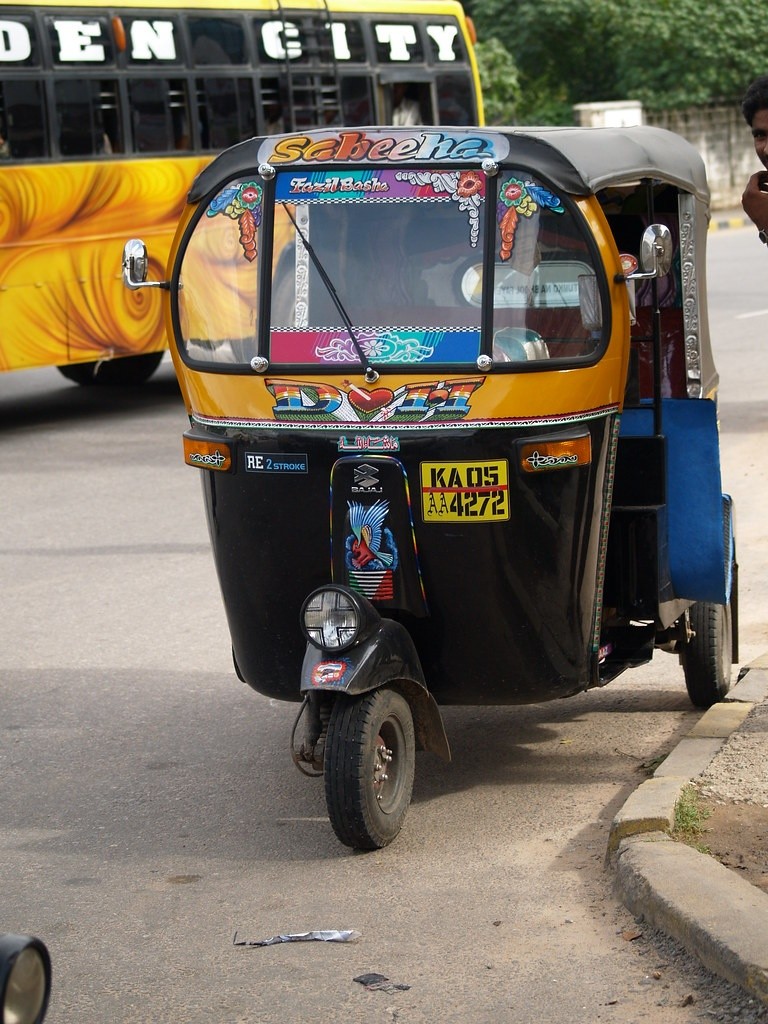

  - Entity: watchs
[758,228,768,244]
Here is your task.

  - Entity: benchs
[603,434,675,611]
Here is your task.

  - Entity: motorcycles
[122,121,739,852]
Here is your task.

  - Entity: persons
[322,96,346,127]
[391,84,423,126]
[171,110,202,150]
[738,74,768,248]
[261,97,284,133]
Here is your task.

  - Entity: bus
[0,0,485,394]
